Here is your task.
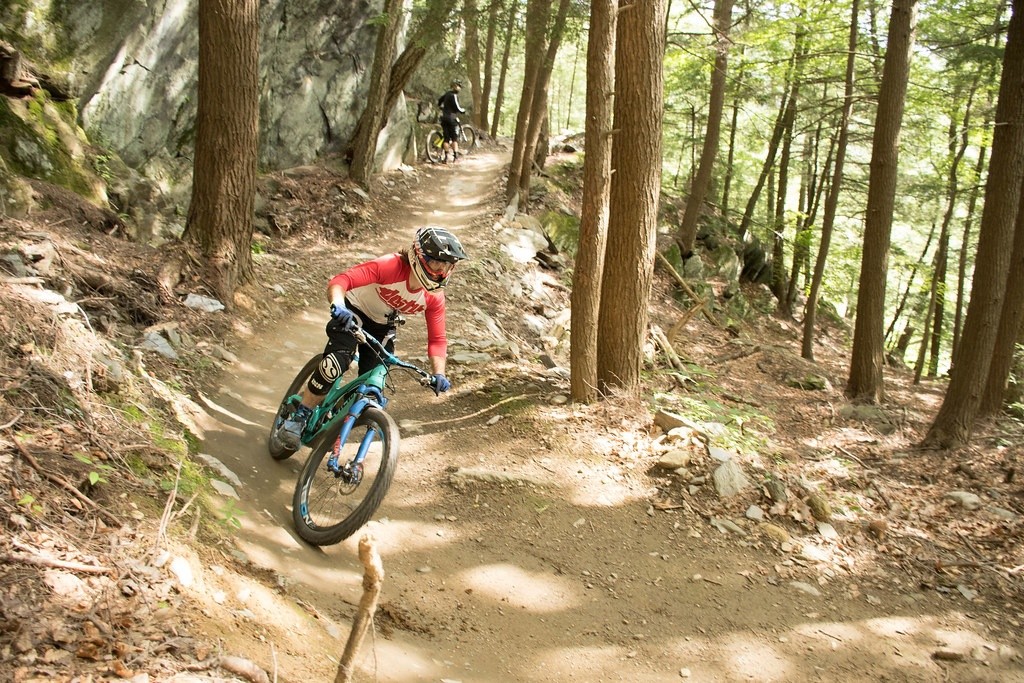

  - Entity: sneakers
[276,405,312,448]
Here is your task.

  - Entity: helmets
[450,78,463,92]
[408,226,468,292]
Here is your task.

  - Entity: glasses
[419,253,455,279]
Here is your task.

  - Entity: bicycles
[270,305,453,546]
[425,107,477,164]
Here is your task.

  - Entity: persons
[273,228,471,446]
[436,77,466,164]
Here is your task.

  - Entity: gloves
[433,373,450,394]
[332,304,354,332]
